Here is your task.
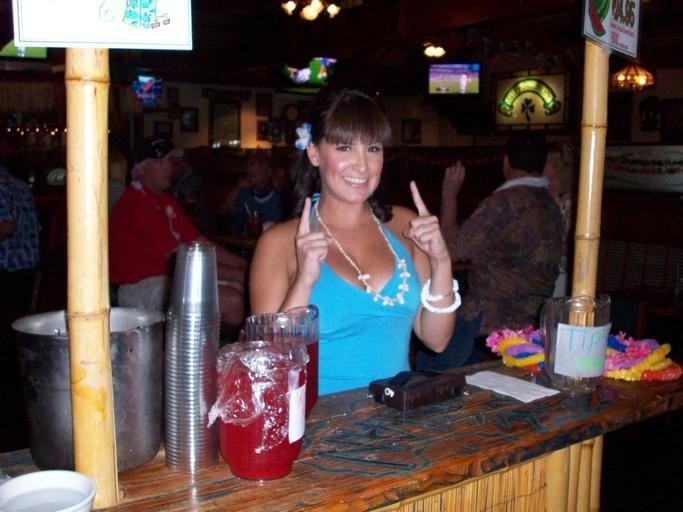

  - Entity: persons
[0,168,40,346]
[287,57,336,83]
[109,133,289,330]
[246,86,462,403]
[415,133,577,372]
[458,72,467,94]
[17,47,25,57]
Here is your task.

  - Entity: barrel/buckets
[11,304,166,475]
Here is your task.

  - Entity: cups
[0,470,96,512]
[540,294,612,398]
[245,215,261,245]
[216,340,310,481]
[163,240,221,474]
[244,305,319,420]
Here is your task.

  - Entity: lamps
[611,58,654,88]
[282,0,341,20]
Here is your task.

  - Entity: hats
[131,134,185,164]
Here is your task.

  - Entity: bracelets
[421,277,462,315]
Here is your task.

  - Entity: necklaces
[314,199,411,306]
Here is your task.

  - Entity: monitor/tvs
[421,60,484,101]
[129,67,168,105]
[275,51,338,95]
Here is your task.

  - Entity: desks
[1,360,683,512]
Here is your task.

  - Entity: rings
[419,224,426,235]
